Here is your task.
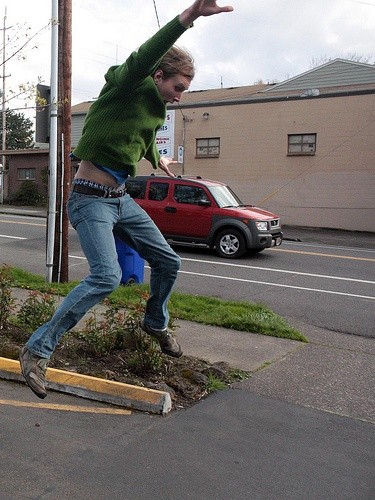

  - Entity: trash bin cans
[113,236,144,286]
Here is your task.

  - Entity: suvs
[121,173,284,258]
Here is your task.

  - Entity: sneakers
[140,319,183,358]
[18,345,49,399]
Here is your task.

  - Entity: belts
[73,184,127,198]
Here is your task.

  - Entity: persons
[19,1,233,399]
